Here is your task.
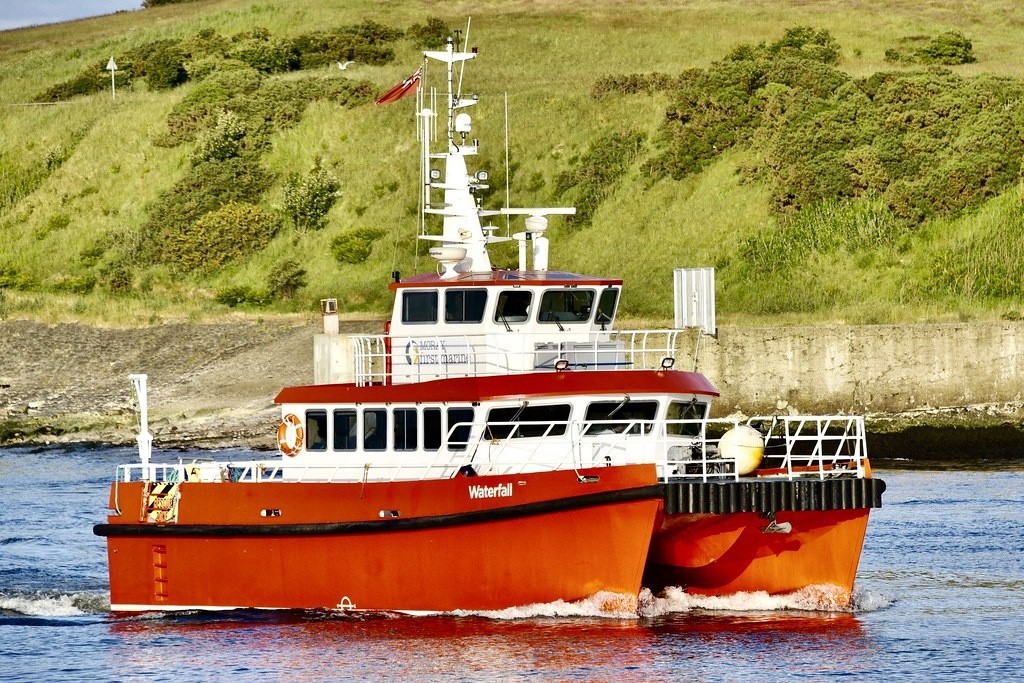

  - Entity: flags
[376,67,422,106]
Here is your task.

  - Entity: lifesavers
[278,413,303,456]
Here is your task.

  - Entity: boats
[93,17,887,619]
